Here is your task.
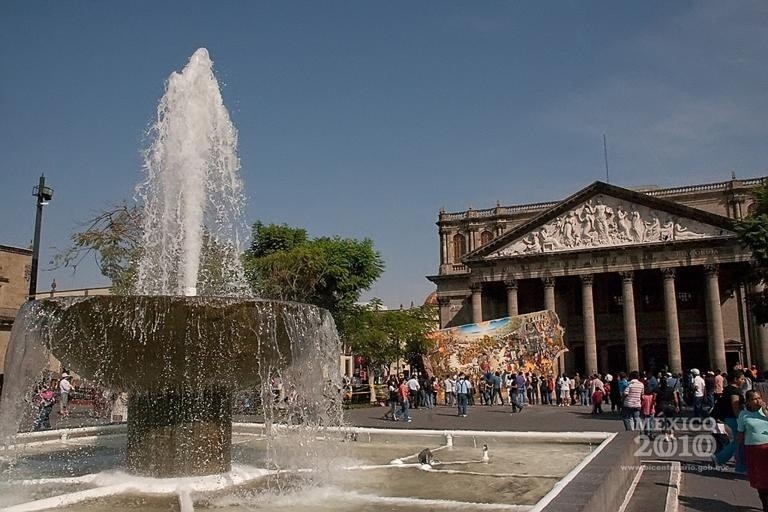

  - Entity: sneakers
[587,404,590,406]
[558,402,569,408]
[384,412,412,422]
[510,406,523,413]
[460,414,467,417]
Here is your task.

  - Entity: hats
[61,373,70,378]
[667,373,672,376]
[691,369,699,374]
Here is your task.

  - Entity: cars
[232,376,390,422]
[54,379,106,412]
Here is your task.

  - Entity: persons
[28,372,128,433]
[514,196,706,256]
[733,388,768,511]
[710,369,747,475]
[708,395,730,471]
[340,360,768,422]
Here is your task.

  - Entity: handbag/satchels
[390,391,398,402]
[42,396,56,407]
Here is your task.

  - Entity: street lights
[30,171,55,299]
